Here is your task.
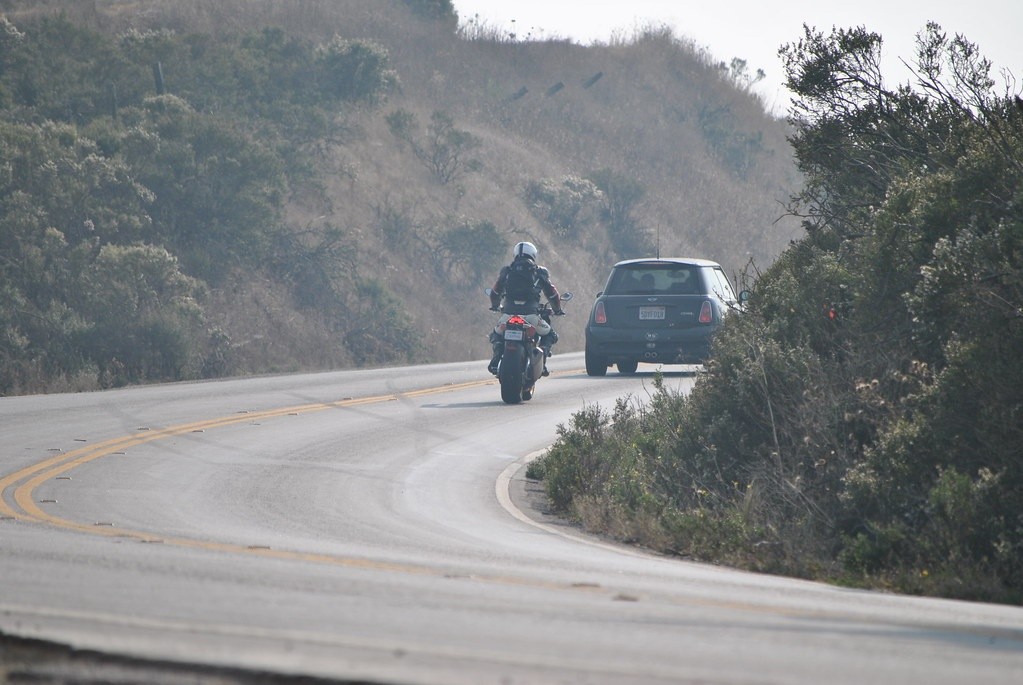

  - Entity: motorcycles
[485,289,574,405]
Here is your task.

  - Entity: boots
[539,342,552,376]
[488,340,504,373]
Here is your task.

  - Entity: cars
[585,257,753,377]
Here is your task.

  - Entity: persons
[488,242,562,376]
[640,273,655,290]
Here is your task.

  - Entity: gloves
[492,304,504,313]
[553,309,565,318]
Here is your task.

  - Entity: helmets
[513,241,537,262]
